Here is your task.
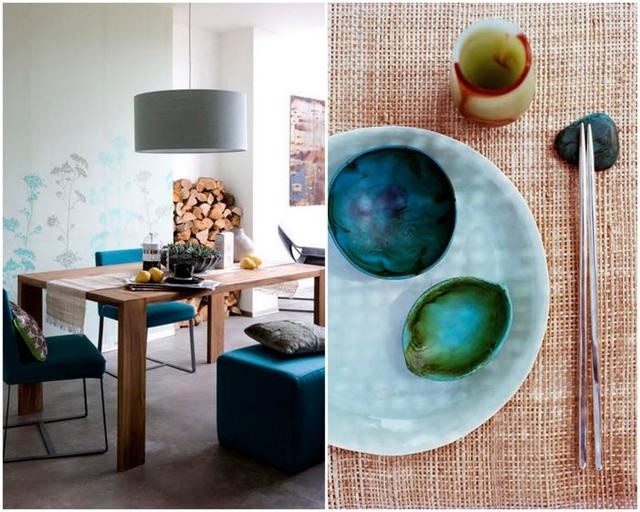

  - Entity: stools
[215,346,325,475]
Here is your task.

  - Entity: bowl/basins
[169,255,223,273]
[400,276,513,386]
[327,146,456,284]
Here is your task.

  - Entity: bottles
[230,227,258,263]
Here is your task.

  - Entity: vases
[235,228,255,262]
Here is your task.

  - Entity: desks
[17,257,325,472]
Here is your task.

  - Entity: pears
[240,255,261,269]
[135,267,164,283]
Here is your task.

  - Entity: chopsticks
[576,123,604,471]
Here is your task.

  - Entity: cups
[158,249,169,271]
[448,17,535,126]
[174,263,194,281]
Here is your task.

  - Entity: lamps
[132,3,249,156]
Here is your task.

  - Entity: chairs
[3,287,111,463]
[278,224,326,314]
[94,247,196,380]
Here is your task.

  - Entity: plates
[327,125,549,459]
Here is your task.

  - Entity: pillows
[9,301,49,362]
[243,317,325,356]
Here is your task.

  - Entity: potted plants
[161,243,223,272]
